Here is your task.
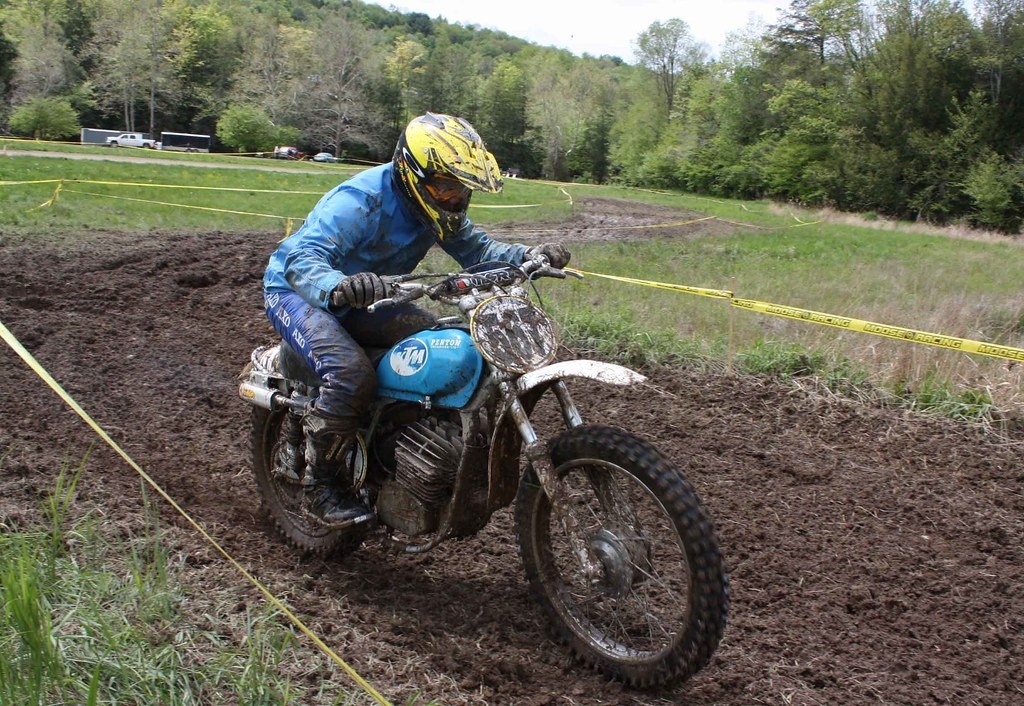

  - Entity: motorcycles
[236,255,732,698]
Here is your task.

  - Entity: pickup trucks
[106,133,156,149]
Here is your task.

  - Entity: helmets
[392,111,504,243]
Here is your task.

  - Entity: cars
[273,146,305,160]
[314,152,337,163]
[155,141,162,149]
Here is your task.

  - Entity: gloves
[523,239,571,279]
[337,271,383,309]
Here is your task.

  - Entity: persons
[263,110,572,530]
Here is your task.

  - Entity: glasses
[428,176,473,195]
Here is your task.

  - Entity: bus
[160,131,212,153]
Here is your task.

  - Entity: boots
[299,426,373,530]
